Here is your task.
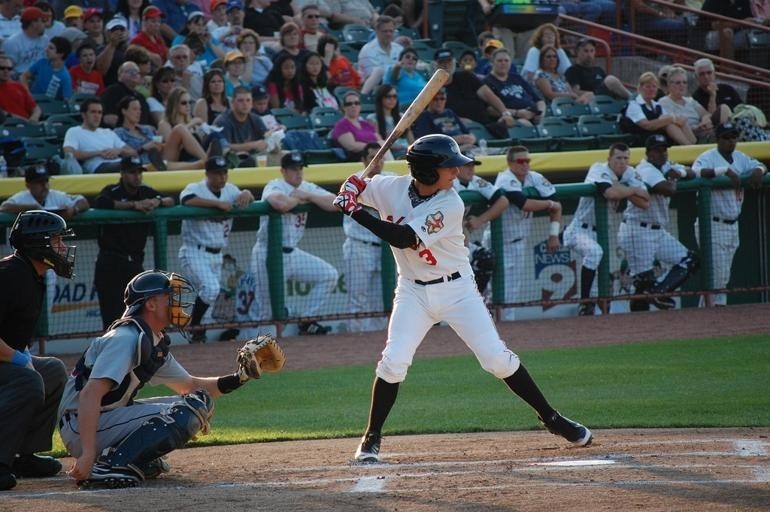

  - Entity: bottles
[479,137,488,157]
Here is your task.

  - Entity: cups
[152,135,163,151]
[253,155,268,167]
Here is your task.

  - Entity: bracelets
[11,349,29,368]
[217,374,242,395]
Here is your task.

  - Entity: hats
[485,39,502,49]
[282,153,305,168]
[27,165,49,182]
[210,0,243,11]
[646,135,670,150]
[224,52,245,66]
[434,49,453,61]
[22,5,164,30]
[120,155,148,173]
[716,123,738,137]
[205,156,229,172]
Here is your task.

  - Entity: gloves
[332,175,366,212]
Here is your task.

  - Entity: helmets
[9,210,76,278]
[406,134,474,167]
[124,268,197,327]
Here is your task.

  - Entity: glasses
[181,101,192,105]
[308,15,320,18]
[344,94,396,106]
[406,55,418,61]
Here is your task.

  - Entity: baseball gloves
[237,335,286,385]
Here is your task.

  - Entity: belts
[198,245,220,254]
[712,217,736,224]
[582,223,596,230]
[282,247,293,252]
[640,223,660,229]
[415,272,461,285]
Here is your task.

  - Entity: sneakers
[578,304,593,316]
[644,290,675,310]
[0,455,62,490]
[545,413,591,446]
[93,460,144,488]
[355,431,381,461]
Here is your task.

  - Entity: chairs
[1,1,768,174]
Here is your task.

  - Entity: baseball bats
[335,68,450,209]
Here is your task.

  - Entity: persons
[57,269,284,491]
[0,2,769,321]
[335,133,592,465]
[0,208,76,491]
